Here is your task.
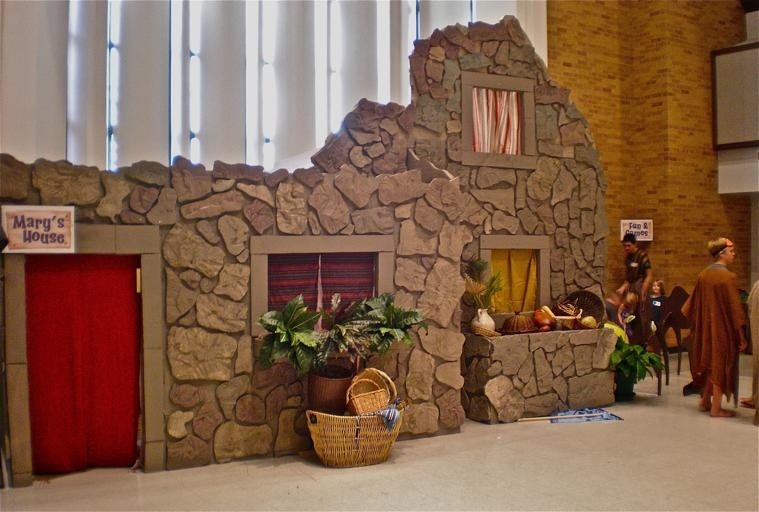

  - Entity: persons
[649,279,666,322]
[614,232,653,351]
[680,237,749,417]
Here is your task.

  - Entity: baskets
[474,327,502,336]
[306,367,409,468]
[554,290,605,329]
[500,315,538,333]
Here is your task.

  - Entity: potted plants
[253,291,429,377]
[608,335,666,401]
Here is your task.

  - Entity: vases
[470,307,496,336]
